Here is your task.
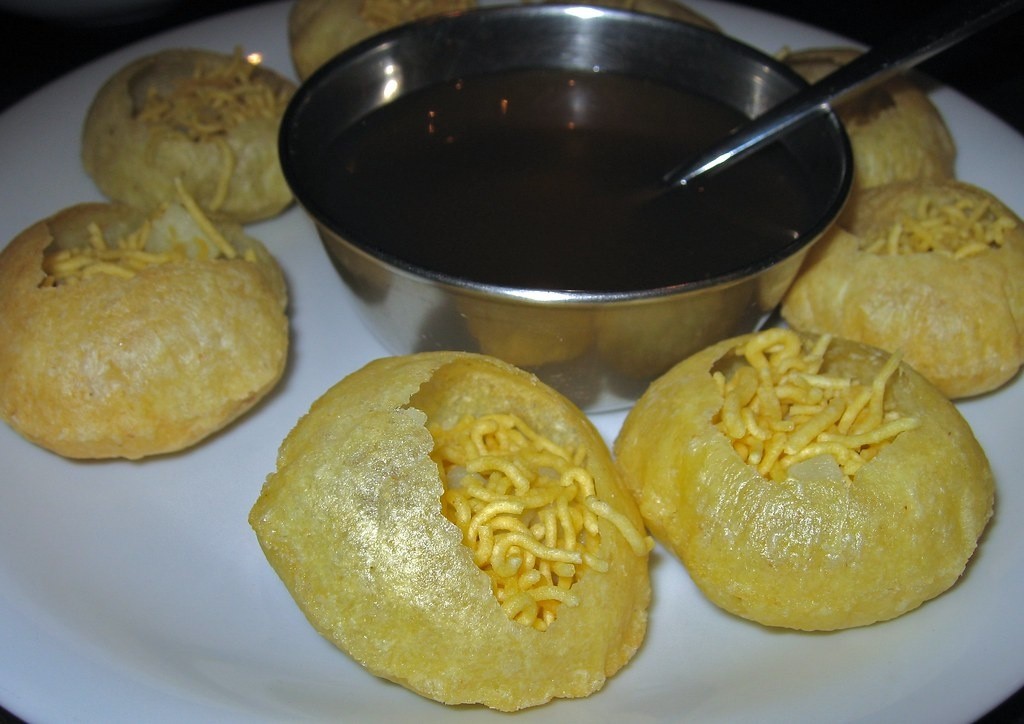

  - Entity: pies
[1,0,1023,711]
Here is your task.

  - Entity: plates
[0,0,1024,724]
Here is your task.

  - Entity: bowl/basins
[277,6,857,417]
